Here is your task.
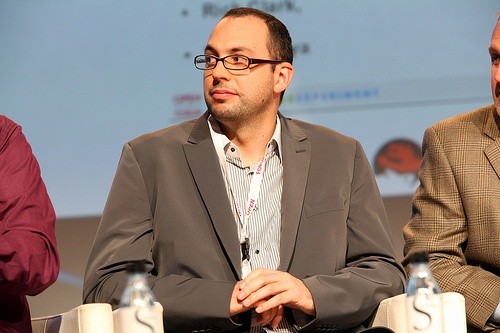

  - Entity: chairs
[30,292,466,333]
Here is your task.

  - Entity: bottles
[120,260,158,333]
[405,251,446,333]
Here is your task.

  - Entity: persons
[402,16,500,333]
[0,113,62,333]
[83,7,409,333]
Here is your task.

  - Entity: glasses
[193,54,286,71]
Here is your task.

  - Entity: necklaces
[208,113,269,280]
